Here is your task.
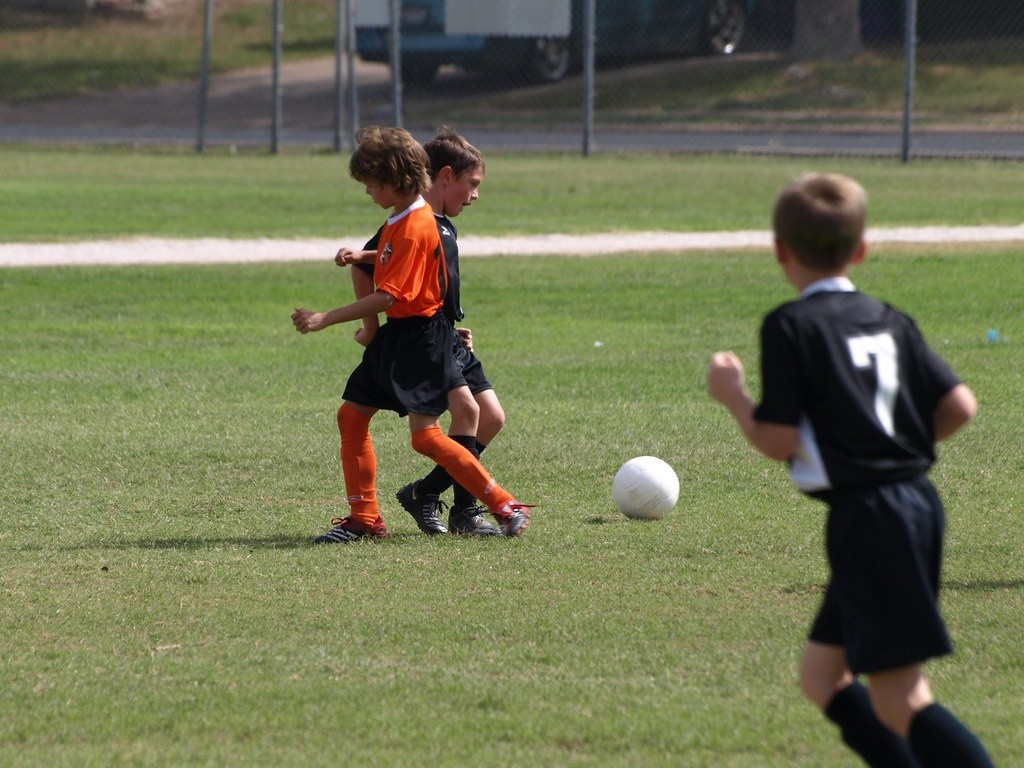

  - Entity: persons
[353,133,505,536]
[292,128,532,545]
[708,175,994,768]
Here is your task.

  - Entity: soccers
[613,455,680,519]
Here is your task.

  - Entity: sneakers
[316,514,390,543]
[396,481,448,534]
[495,501,531,538]
[448,507,502,537]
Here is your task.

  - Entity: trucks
[353,1,1022,87]
